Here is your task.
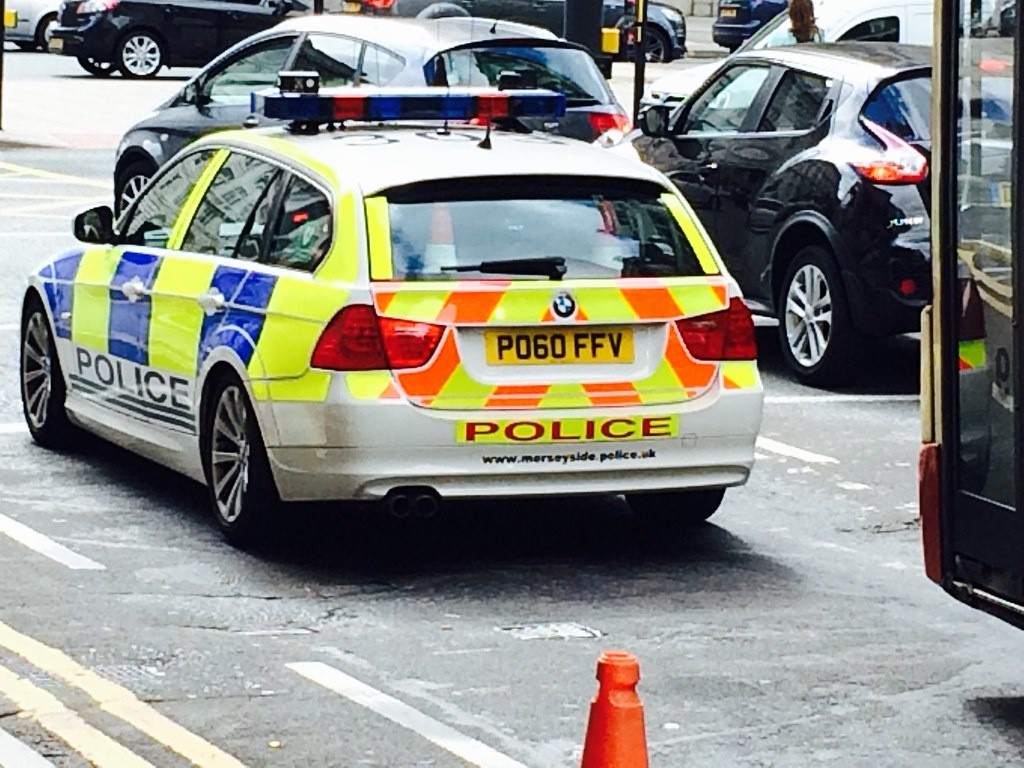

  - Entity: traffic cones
[421,204,461,278]
[578,648,656,768]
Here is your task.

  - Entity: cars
[642,39,1014,387]
[19,65,762,542]
[1,0,686,78]
[724,1,1016,120]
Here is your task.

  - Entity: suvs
[110,14,637,229]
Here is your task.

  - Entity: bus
[912,0,1024,635]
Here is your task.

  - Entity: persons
[788,0,818,43]
